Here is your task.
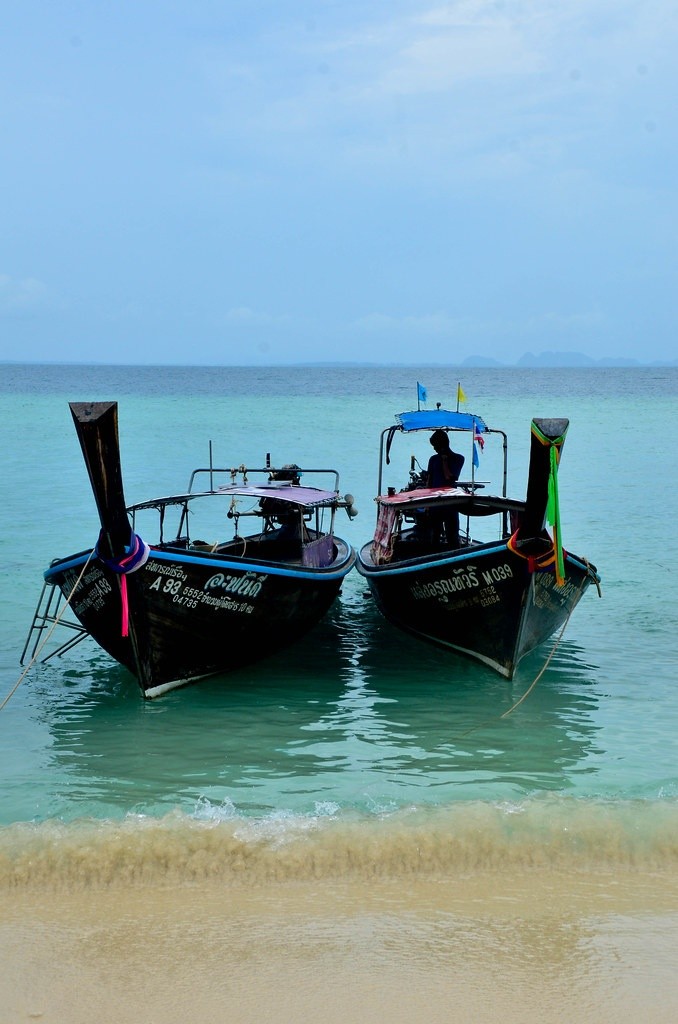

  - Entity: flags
[416,381,427,401]
[457,382,465,402]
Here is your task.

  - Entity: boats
[353,380,600,683]
[41,399,359,702]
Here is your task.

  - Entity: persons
[418,431,465,549]
[259,463,304,559]
